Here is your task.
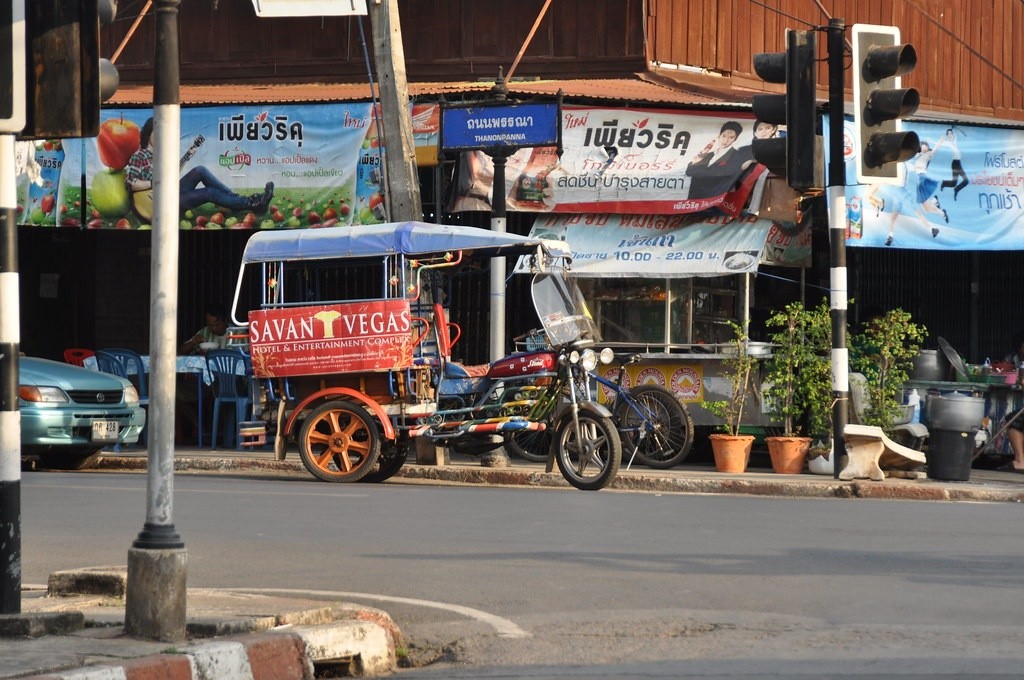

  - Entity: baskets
[526,335,546,351]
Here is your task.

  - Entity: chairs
[65,349,95,368]
[203,349,257,450]
[95,351,149,452]
[847,373,929,456]
[110,349,148,450]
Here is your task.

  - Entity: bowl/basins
[893,404,915,424]
[200,342,219,350]
[993,372,1018,384]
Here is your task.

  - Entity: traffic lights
[749,28,821,191]
[854,24,922,181]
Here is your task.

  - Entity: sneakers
[251,181,274,215]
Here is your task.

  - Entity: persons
[868,129,969,246]
[686,120,780,200]
[997,344,1024,474]
[125,117,274,216]
[180,303,249,445]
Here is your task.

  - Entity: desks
[891,379,1023,455]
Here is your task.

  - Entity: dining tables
[83,355,260,452]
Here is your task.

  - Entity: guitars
[130,133,206,225]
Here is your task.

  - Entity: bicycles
[509,333,694,467]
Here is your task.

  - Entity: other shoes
[942,209,948,223]
[954,188,957,201]
[877,206,879,217]
[932,228,939,237]
[885,236,893,246]
[934,195,940,208]
[880,199,884,211]
[941,181,944,191]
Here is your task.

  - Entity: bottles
[24,139,65,227]
[908,389,920,423]
[982,363,991,374]
[973,366,978,375]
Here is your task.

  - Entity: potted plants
[808,303,929,475]
[760,300,821,475]
[701,319,755,472]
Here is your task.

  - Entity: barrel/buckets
[927,429,977,480]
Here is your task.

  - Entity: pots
[908,348,954,381]
[926,387,985,433]
[721,342,771,354]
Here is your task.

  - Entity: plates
[724,253,755,271]
[970,375,1007,383]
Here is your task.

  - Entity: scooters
[230,225,622,490]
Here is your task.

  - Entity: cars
[17,348,147,472]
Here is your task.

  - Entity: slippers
[995,461,1024,474]
